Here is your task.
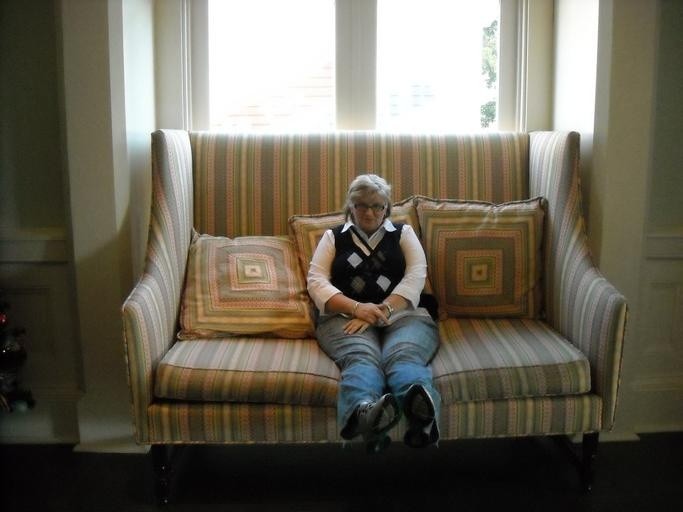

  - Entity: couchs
[118,128,628,448]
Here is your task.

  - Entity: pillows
[178,221,317,340]
[285,197,438,329]
[411,194,548,324]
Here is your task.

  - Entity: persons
[306,173,440,451]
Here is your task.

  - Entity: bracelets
[350,302,359,318]
[379,301,392,321]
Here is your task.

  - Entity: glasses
[349,202,388,212]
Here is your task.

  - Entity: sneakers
[353,392,400,454]
[400,381,441,449]
